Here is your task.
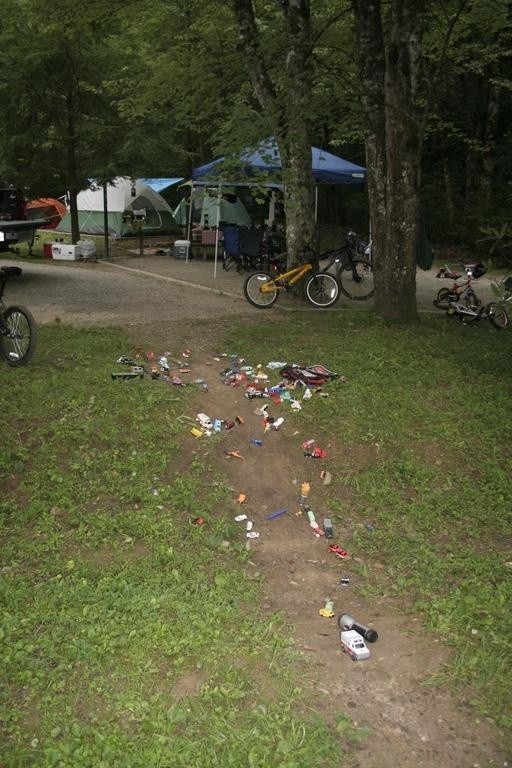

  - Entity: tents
[53,175,184,238]
[173,187,253,230]
[23,196,67,230]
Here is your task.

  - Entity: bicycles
[434,299,510,329]
[268,230,375,301]
[244,243,340,308]
[0,265,37,367]
[335,230,370,270]
[437,259,488,310]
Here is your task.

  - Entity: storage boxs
[202,229,223,245]
[174,240,190,259]
[43,242,60,257]
[52,244,80,261]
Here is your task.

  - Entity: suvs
[0,188,52,256]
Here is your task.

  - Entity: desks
[190,243,216,260]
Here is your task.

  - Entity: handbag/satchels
[280,364,339,385]
